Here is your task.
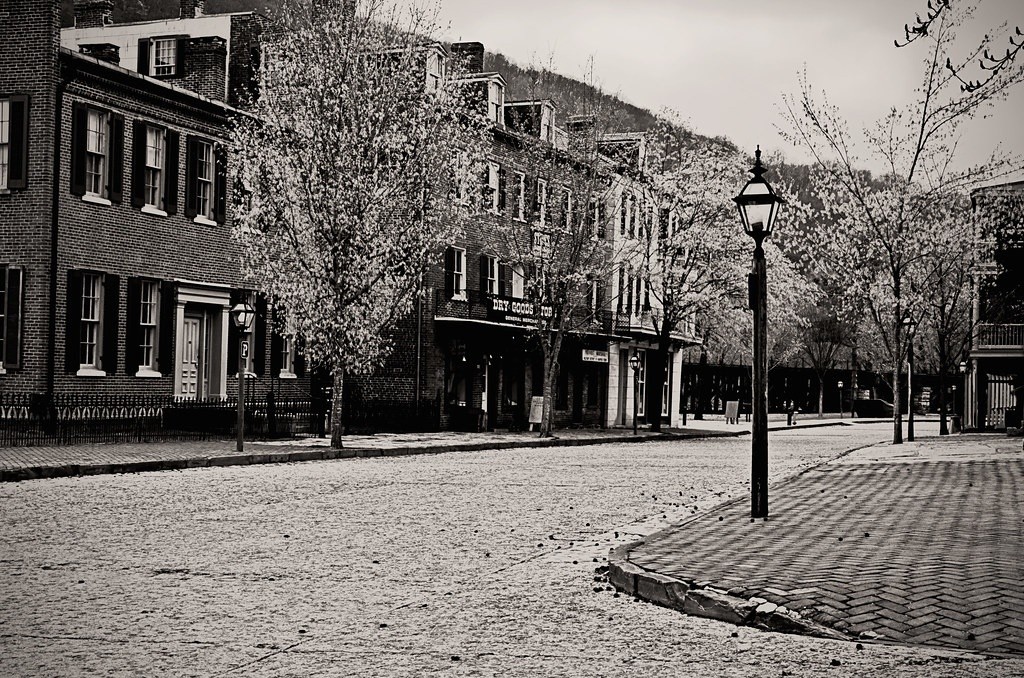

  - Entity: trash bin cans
[948,416,961,434]
[725,400,743,424]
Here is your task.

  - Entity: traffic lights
[850,372,859,388]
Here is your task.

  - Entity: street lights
[951,385,957,415]
[837,380,844,418]
[903,310,917,441]
[628,356,643,435]
[730,145,789,519]
[228,294,256,453]
